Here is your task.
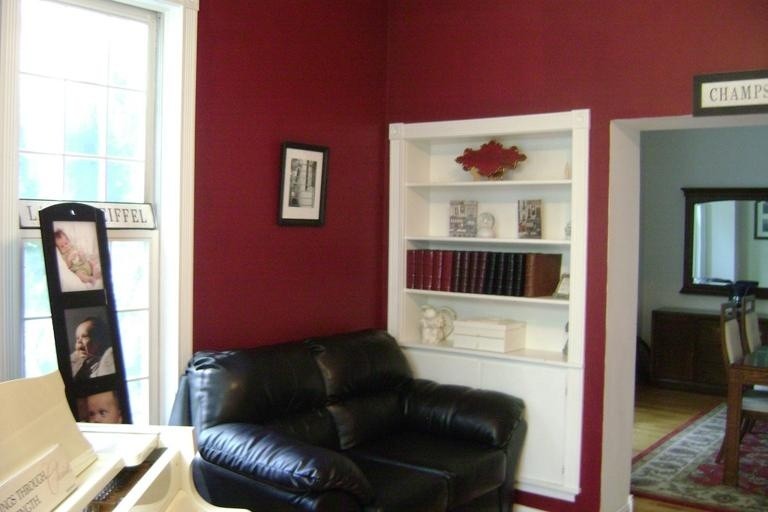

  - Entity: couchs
[168,328,526,512]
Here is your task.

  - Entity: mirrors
[680,186,768,300]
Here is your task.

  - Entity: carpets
[631,400,768,512]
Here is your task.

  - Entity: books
[405,250,563,297]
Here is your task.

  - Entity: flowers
[454,139,526,176]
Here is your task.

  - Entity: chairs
[713,302,768,464]
[739,294,768,442]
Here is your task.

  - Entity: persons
[85,389,127,423]
[54,229,100,285]
[72,316,118,379]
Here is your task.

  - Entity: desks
[722,346,768,486]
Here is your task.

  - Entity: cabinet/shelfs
[386,109,591,502]
[651,305,768,396]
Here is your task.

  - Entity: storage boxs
[452,320,526,353]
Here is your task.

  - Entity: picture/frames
[753,201,768,239]
[277,141,328,227]
